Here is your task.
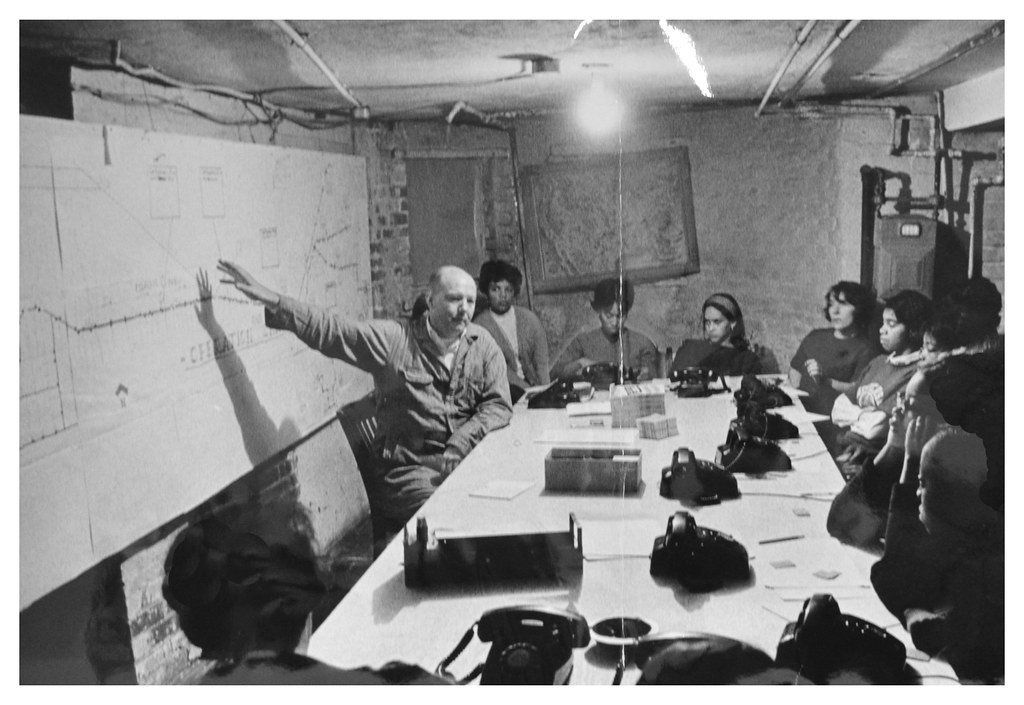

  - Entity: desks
[306,371,963,685]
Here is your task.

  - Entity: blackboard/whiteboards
[19,113,380,617]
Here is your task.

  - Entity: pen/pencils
[758,534,805,544]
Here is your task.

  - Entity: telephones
[659,446,740,507]
[583,362,621,391]
[475,604,591,686]
[715,418,792,474]
[735,374,793,408]
[669,363,720,398]
[649,511,749,594]
[775,592,906,684]
[741,399,799,439]
[528,376,581,409]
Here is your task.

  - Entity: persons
[870,427,1005,685]
[912,308,993,370]
[550,277,658,388]
[825,358,1005,560]
[826,289,938,478]
[158,497,454,686]
[666,293,763,381]
[937,271,1003,343]
[788,279,879,445]
[216,257,515,530]
[471,260,550,403]
